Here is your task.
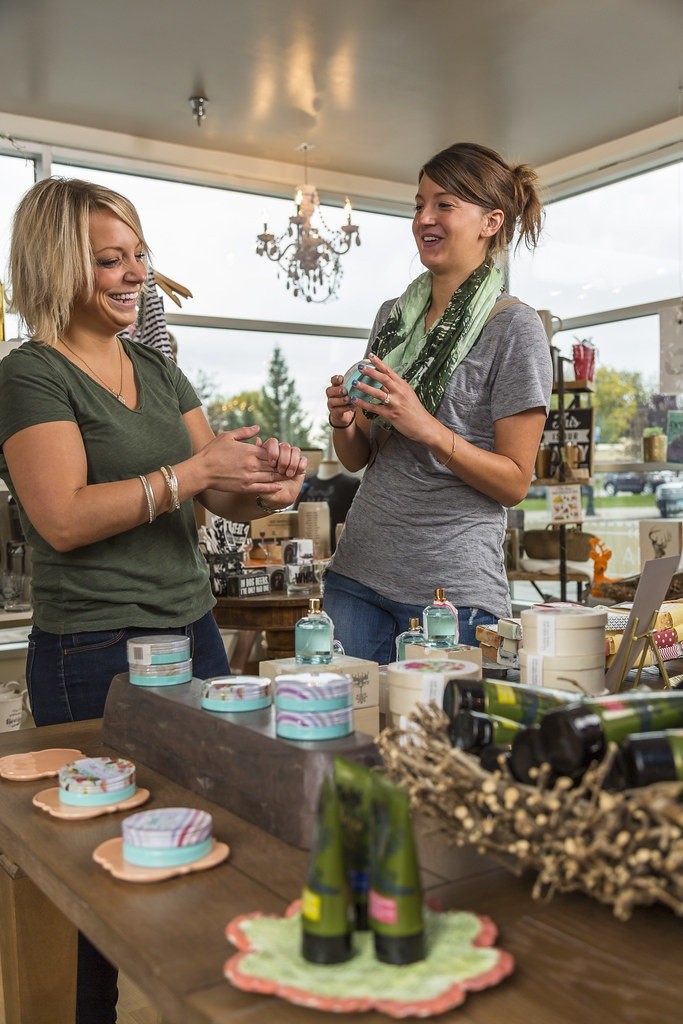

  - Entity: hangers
[153,268,193,309]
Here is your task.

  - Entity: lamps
[256,142,361,305]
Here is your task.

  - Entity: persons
[0,180,306,728]
[321,143,553,666]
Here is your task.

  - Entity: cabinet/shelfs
[507,348,596,605]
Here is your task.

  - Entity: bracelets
[329,410,355,428]
[138,475,156,524]
[255,495,294,513]
[160,465,180,513]
[438,430,455,467]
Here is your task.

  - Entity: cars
[601,458,679,498]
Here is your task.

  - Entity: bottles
[394,617,423,661]
[422,589,458,648]
[294,599,334,662]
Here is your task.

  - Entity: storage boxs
[251,511,298,539]
[226,574,272,597]
[638,519,683,574]
[258,642,482,738]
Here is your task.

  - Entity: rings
[382,393,390,405]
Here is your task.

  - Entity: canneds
[297,500,330,559]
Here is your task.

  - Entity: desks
[0,716,683,1023]
[211,585,323,677]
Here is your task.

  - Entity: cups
[0,676,35,733]
[573,346,595,382]
[285,564,313,594]
[0,574,32,614]
[536,443,559,480]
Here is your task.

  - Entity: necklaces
[58,336,126,406]
